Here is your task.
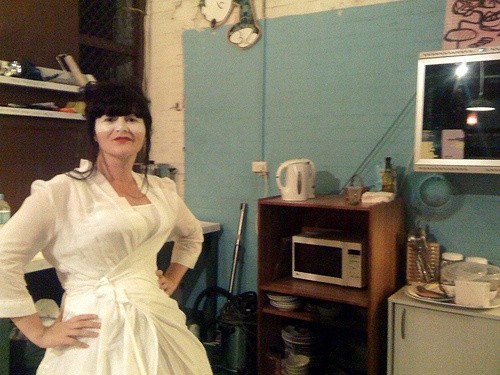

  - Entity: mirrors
[412,51,500,174]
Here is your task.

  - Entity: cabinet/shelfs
[257,193,405,375]
[0,0,146,218]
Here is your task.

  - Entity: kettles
[275,158,314,201]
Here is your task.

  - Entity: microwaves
[291,234,366,288]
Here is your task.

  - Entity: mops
[189,200,261,375]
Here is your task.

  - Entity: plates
[405,284,500,309]
[267,292,300,311]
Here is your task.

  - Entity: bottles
[0,194,11,232]
[382,157,394,193]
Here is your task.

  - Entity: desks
[0,220,221,375]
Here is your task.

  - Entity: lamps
[465,61,496,112]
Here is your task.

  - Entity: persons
[0,79,204,374]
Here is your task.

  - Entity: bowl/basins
[285,355,310,375]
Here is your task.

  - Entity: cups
[348,187,361,205]
[454,281,490,307]
